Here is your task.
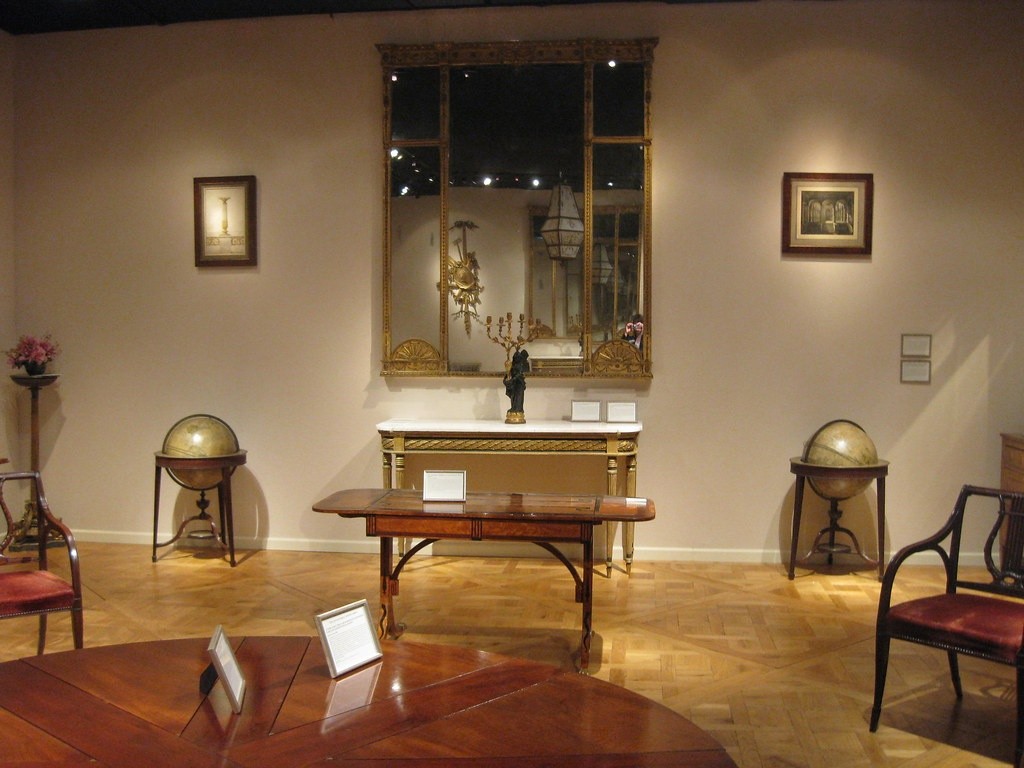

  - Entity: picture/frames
[193,175,257,268]
[782,173,874,255]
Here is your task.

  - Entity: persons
[503,352,526,413]
[520,350,530,372]
[621,314,645,352]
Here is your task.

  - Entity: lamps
[590,238,614,284]
[539,167,585,259]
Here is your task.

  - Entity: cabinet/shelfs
[996,433,1024,568]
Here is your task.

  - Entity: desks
[312,489,656,676]
[376,417,643,578]
[10,375,60,527]
[0,635,746,768]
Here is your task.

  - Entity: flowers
[1,333,62,369]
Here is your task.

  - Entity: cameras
[631,324,638,329]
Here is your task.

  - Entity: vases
[23,364,46,375]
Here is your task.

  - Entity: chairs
[0,457,83,650]
[870,485,1024,768]
